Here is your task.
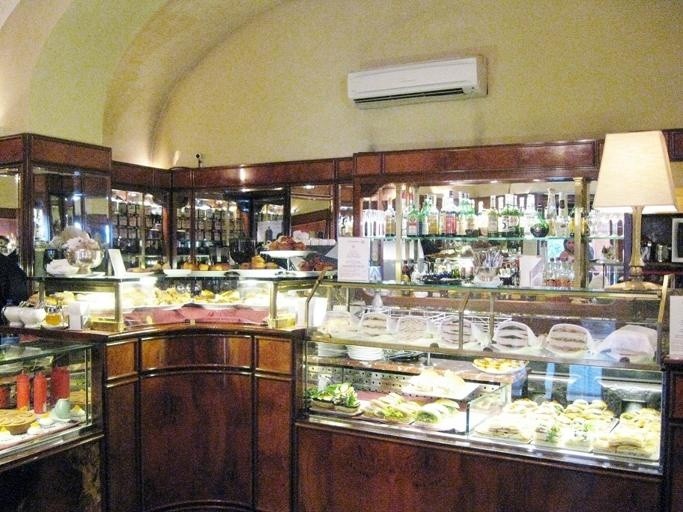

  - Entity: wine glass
[65,248,105,274]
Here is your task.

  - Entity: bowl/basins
[18,308,47,329]
[0,412,39,436]
[3,306,25,328]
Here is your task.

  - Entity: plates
[470,358,529,375]
[305,397,460,433]
[401,377,481,402]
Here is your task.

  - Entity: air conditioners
[347,55,487,106]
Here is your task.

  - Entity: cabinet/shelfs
[0,159,682,512]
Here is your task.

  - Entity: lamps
[592,130,677,293]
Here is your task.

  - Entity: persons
[557,238,574,262]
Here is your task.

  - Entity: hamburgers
[310,392,460,426]
[506,398,662,432]
[409,369,465,394]
[488,416,661,458]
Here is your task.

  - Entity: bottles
[542,254,575,289]
[33,370,47,414]
[16,368,31,411]
[55,397,72,419]
[359,188,575,238]
